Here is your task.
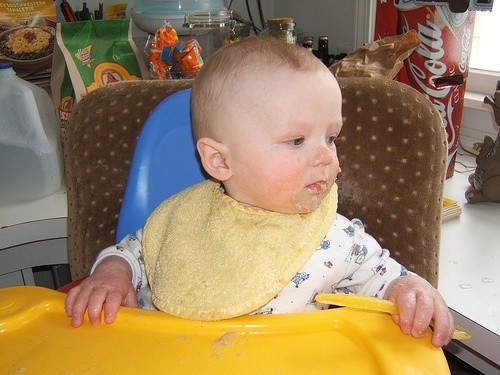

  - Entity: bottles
[0,63,66,209]
[301,35,314,54]
[318,36,331,68]
[268,17,298,45]
[183,8,236,63]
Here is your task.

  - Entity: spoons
[316,294,473,340]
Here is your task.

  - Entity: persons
[65,37,454,347]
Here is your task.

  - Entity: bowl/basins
[0,24,57,70]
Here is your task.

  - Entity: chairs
[64,72,500,375]
